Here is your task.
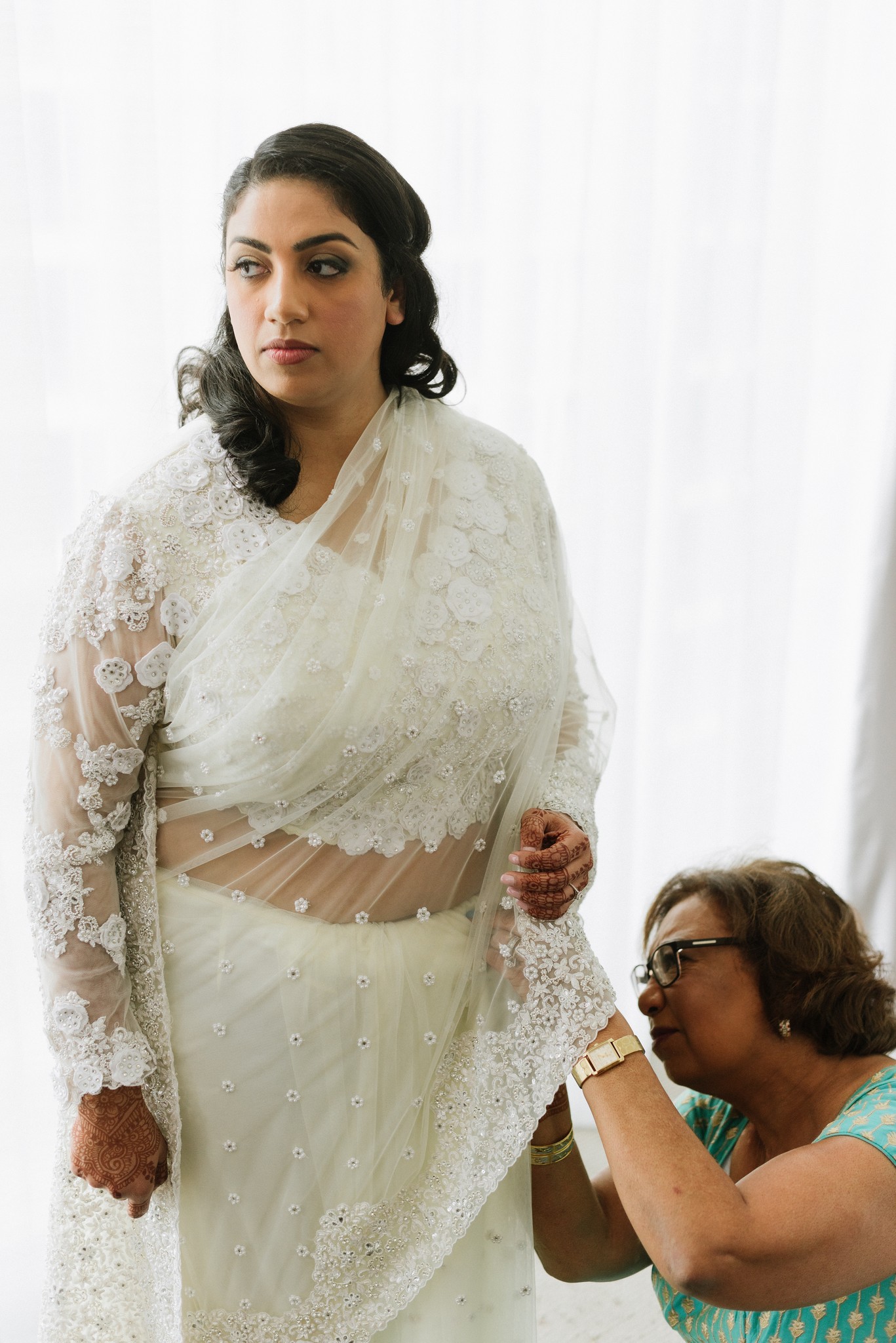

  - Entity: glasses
[628,934,745,1001]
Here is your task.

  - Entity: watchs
[529,1125,574,1165]
[567,1031,648,1089]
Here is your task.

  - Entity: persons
[12,124,608,1343]
[484,851,896,1342]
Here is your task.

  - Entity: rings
[567,882,584,902]
[500,935,521,967]
